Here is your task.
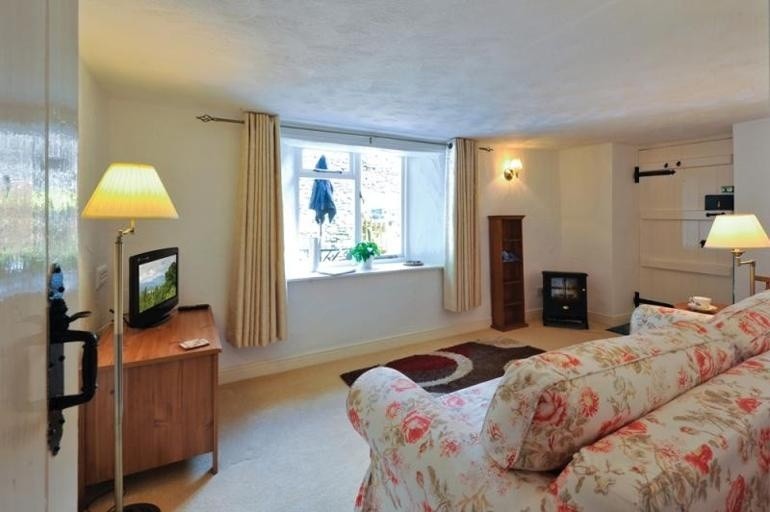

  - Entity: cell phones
[179,338,210,350]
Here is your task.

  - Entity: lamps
[79,162,180,512]
[703,214,770,296]
[503,160,524,181]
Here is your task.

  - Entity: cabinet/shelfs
[542,271,588,329]
[488,215,529,332]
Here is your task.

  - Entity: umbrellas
[307,153,337,263]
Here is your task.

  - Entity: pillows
[714,290,769,362]
[480,318,735,472]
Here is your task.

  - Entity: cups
[685,303,718,312]
[687,295,713,307]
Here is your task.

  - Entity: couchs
[346,305,769,512]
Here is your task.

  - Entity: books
[318,265,356,277]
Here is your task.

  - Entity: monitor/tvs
[129,247,178,330]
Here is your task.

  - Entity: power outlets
[96,264,109,288]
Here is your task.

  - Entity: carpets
[339,335,549,399]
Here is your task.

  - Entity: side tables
[675,303,729,315]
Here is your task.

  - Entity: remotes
[178,303,209,311]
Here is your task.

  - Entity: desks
[78,305,223,485]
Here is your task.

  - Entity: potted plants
[346,242,380,269]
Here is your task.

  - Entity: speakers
[705,194,734,210]
